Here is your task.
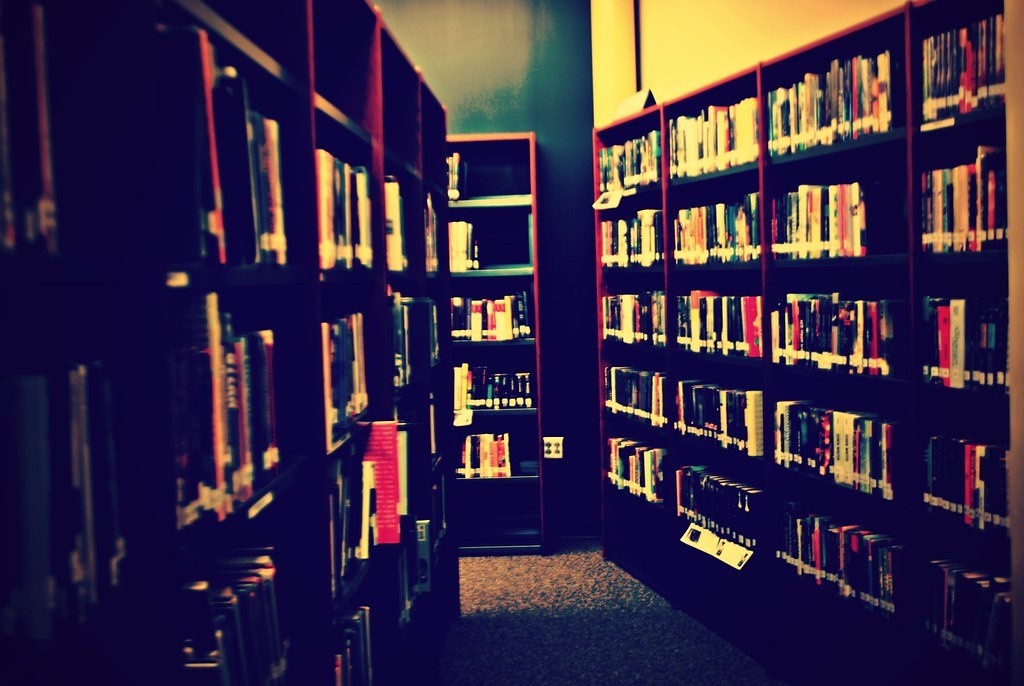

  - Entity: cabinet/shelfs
[0,0,1024,686]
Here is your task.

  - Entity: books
[0,1,536,686]
[594,16,1011,674]
